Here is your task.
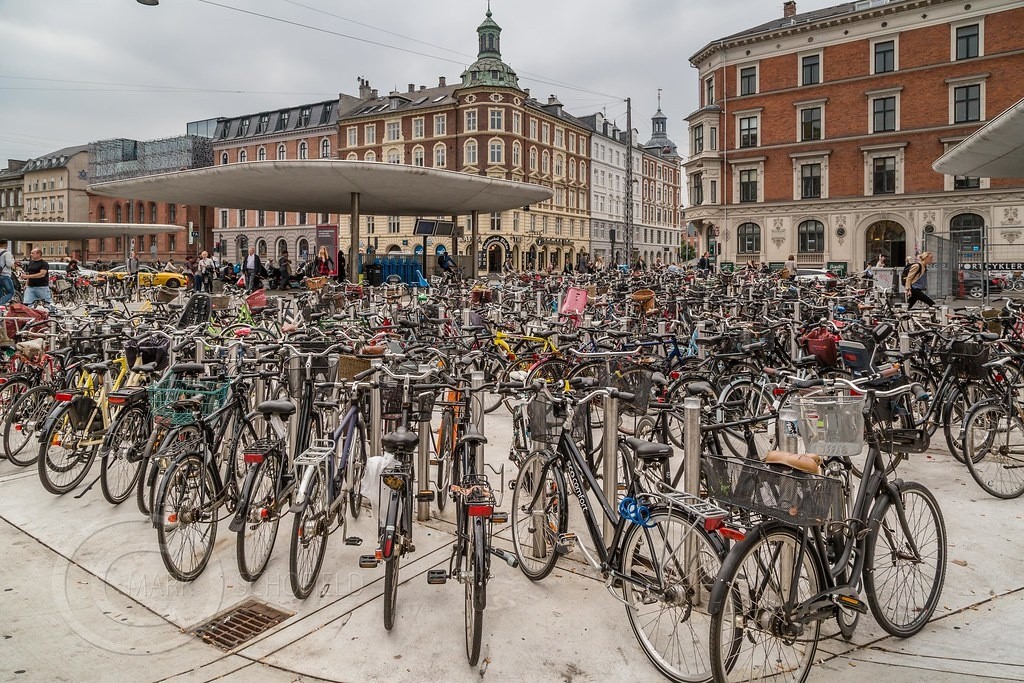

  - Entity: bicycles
[0,266,1024,683]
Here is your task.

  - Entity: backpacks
[901,262,922,287]
[438,255,446,265]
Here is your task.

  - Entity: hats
[0,239,7,244]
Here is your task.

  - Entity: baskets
[379,374,440,422]
[471,290,490,301]
[631,289,654,312]
[598,358,653,415]
[126,340,169,374]
[147,379,231,426]
[323,294,342,309]
[789,396,867,456]
[307,276,328,290]
[155,286,180,303]
[702,455,842,525]
[529,393,586,444]
[980,309,1002,337]
[283,356,339,398]
[212,296,231,310]
[937,341,989,379]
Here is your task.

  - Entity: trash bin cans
[364,264,383,287]
[359,272,367,285]
[872,267,899,297]
[894,267,908,293]
[926,267,951,296]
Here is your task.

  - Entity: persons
[784,254,798,281]
[21,248,54,307]
[440,251,457,277]
[860,252,941,312]
[0,239,15,305]
[59,245,346,294]
[503,258,516,275]
[547,251,710,276]
[747,260,768,270]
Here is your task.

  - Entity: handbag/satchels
[245,288,268,314]
[237,274,246,286]
[805,327,838,367]
[6,304,47,341]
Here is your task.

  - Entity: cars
[96,265,187,288]
[48,263,99,286]
[958,269,1005,298]
[795,269,838,284]
